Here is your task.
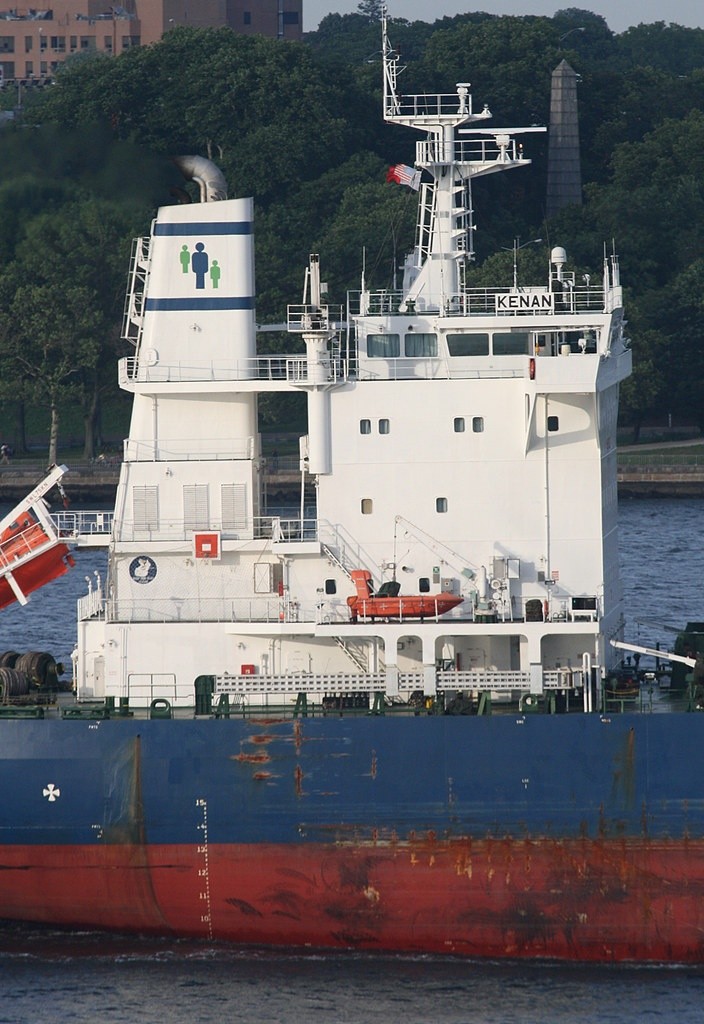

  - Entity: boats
[1,15,704,967]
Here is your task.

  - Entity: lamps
[578,339,588,354]
